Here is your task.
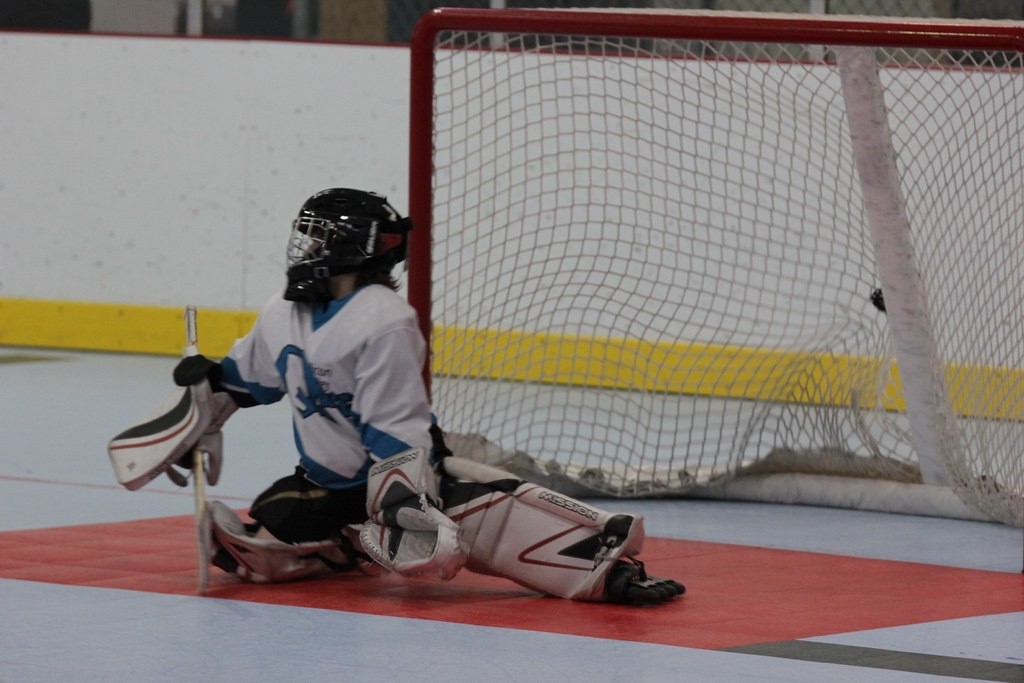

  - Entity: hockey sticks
[185,305,211,593]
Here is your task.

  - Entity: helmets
[280,188,410,301]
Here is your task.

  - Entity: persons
[107,186,688,608]
